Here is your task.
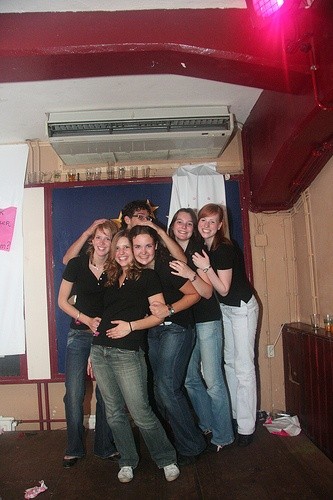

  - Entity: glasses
[129,215,152,222]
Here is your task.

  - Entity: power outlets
[267,344,275,358]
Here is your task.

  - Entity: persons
[57,220,120,469]
[127,225,207,467]
[160,208,234,456]
[61,199,188,266]
[87,228,180,482]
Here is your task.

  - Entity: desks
[282,320,333,466]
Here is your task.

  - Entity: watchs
[203,266,211,273]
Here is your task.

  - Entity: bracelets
[75,312,81,321]
[129,322,132,332]
[166,304,174,315]
[190,273,197,281]
[192,203,259,447]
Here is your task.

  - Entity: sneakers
[118,466,133,482]
[163,465,180,481]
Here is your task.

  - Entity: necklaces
[90,263,106,270]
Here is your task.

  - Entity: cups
[324,313,333,332]
[309,313,320,329]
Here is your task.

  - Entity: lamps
[244,0,303,32]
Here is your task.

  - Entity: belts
[160,321,172,326]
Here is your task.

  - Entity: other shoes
[101,453,120,464]
[238,434,252,446]
[62,456,80,468]
[177,448,206,464]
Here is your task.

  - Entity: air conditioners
[45,104,236,167]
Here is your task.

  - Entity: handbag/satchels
[262,413,301,436]
